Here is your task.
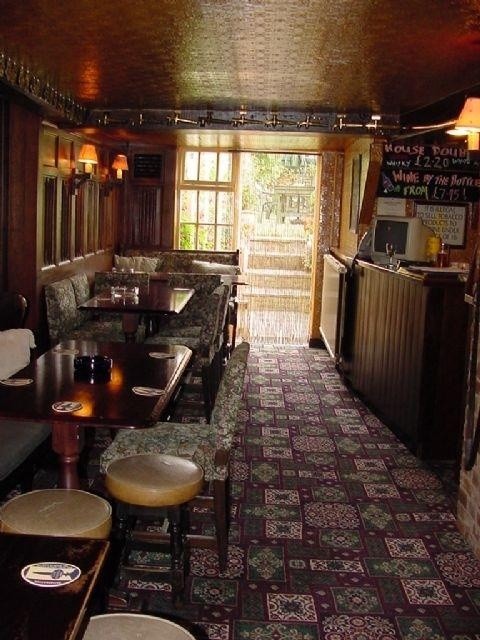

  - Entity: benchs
[115,248,240,349]
[1,418,52,500]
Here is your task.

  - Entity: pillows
[113,254,158,272]
[191,260,240,276]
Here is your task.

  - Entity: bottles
[437,242,449,269]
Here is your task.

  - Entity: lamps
[78,145,98,173]
[111,155,128,180]
[444,97,480,151]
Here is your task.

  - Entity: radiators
[319,253,348,369]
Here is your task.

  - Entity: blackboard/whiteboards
[376,141,480,204]
[414,201,468,250]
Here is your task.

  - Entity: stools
[0,490,114,542]
[104,455,204,606]
[80,611,207,640]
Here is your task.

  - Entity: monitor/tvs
[372,215,435,265]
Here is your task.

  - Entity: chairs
[167,272,220,318]
[100,343,250,574]
[94,273,149,297]
[44,272,144,343]
[145,287,232,422]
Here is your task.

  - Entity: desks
[78,287,196,342]
[1,536,109,640]
[0,340,193,490]
